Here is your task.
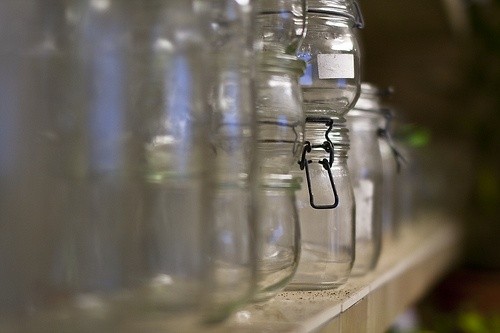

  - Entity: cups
[0,0,405,332]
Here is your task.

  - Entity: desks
[1,221,464,333]
